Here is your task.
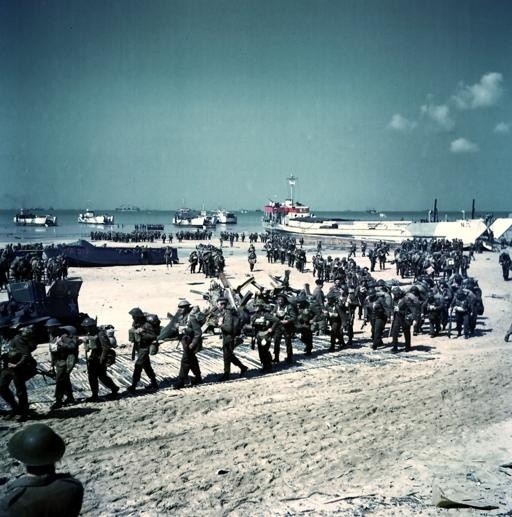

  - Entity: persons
[0,424,83,517]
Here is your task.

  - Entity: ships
[260,171,511,246]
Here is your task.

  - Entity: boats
[77,207,115,225]
[116,201,141,215]
[14,206,58,227]
[172,198,238,229]
[43,239,179,267]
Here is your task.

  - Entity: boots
[128,383,157,389]
[87,386,118,402]
[50,398,74,408]
[221,367,247,378]
[174,377,202,387]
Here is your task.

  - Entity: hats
[368,288,376,296]
[45,320,59,327]
[130,308,142,318]
[8,423,65,466]
[82,319,95,327]
[178,301,190,307]
